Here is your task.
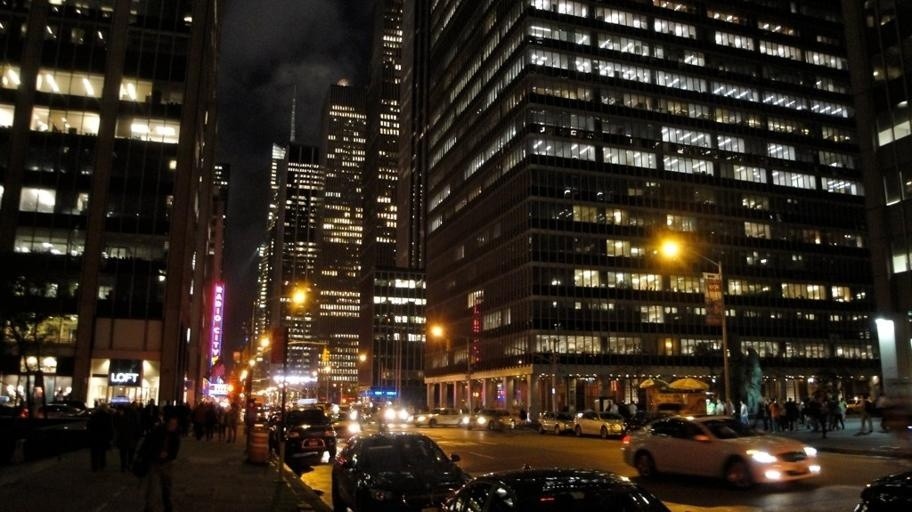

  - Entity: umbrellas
[670,379,709,391]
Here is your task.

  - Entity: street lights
[245,286,309,424]
[653,234,731,416]
[429,324,472,416]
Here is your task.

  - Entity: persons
[606,399,638,419]
[85,398,258,512]
[705,394,874,438]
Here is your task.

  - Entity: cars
[571,408,625,438]
[331,428,472,510]
[852,468,912,511]
[620,412,821,488]
[439,462,670,512]
[18,401,91,431]
[537,412,573,435]
[270,407,337,470]
[475,407,515,431]
[414,407,463,427]
